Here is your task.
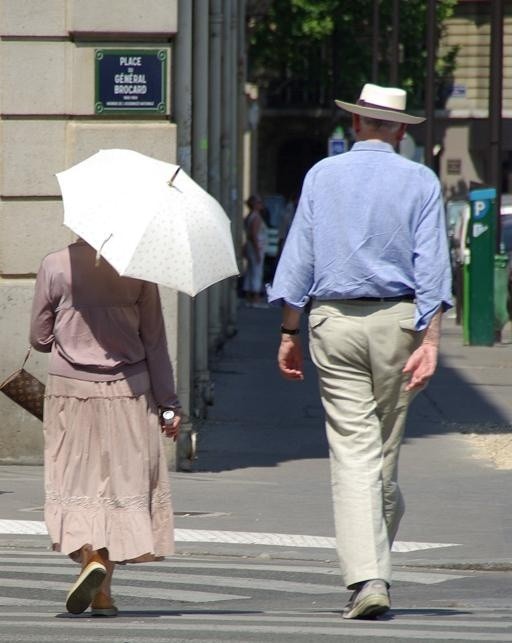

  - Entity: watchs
[280,322,300,335]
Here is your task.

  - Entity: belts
[346,293,416,302]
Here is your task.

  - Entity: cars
[444,193,512,270]
[248,195,290,264]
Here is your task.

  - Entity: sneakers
[66,554,119,617]
[344,580,390,620]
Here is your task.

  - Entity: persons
[264,82,455,621]
[29,237,182,616]
[243,196,272,310]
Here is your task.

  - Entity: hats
[334,84,427,124]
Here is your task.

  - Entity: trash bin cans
[453,249,510,331]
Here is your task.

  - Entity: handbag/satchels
[0,369,45,421]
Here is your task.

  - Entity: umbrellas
[52,148,241,300]
